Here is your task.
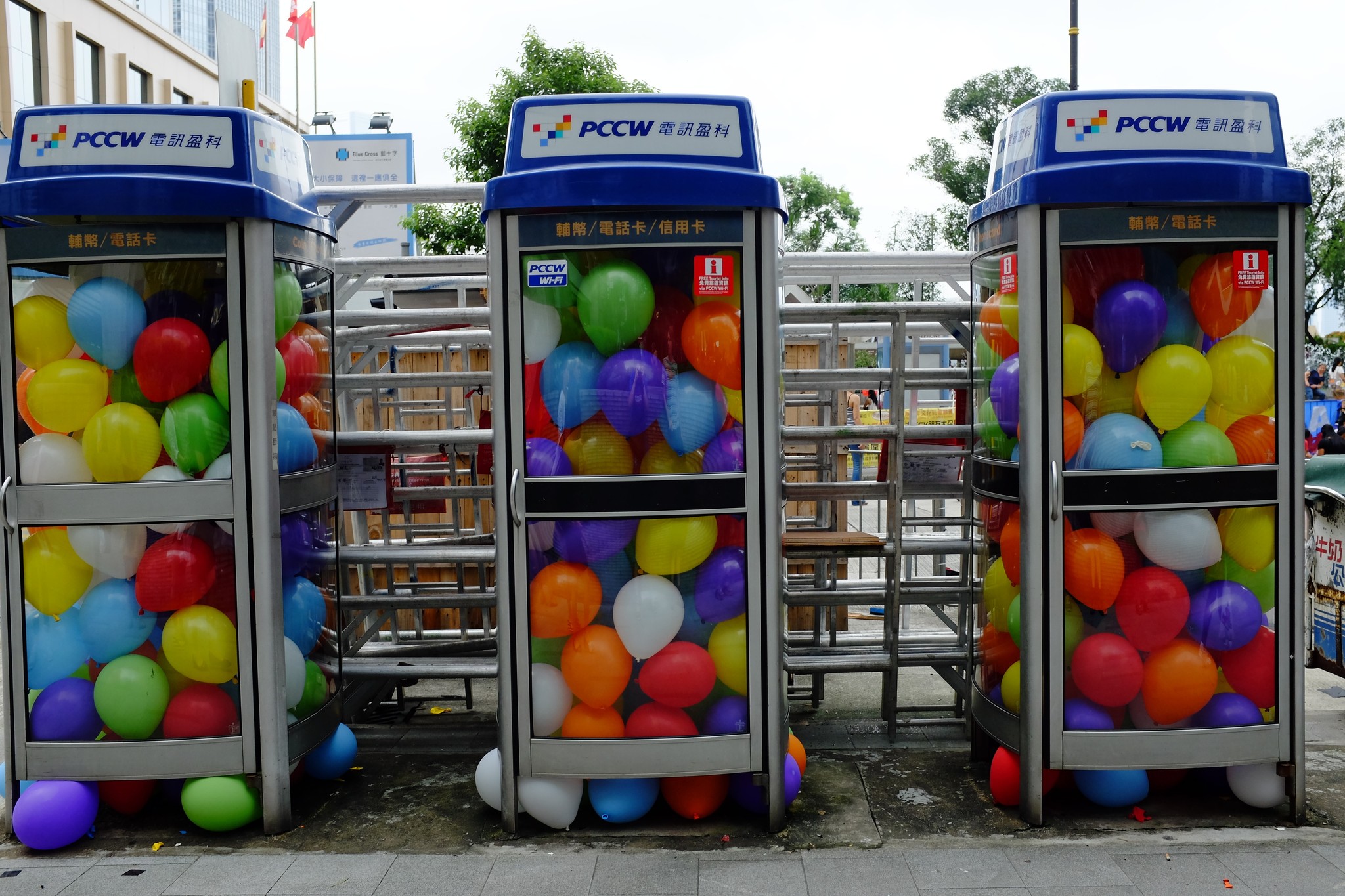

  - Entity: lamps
[369,111,393,134]
[310,111,338,135]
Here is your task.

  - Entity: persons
[846,390,868,506]
[1329,357,1345,400]
[1304,400,1345,458]
[1304,364,1325,400]
[863,389,878,412]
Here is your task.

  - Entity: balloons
[969,247,1292,807]
[473,246,807,832]
[0,261,358,850]
